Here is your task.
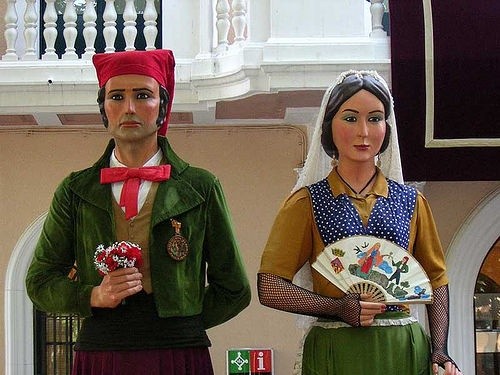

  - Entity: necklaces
[335,165,378,194]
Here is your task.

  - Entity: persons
[26,48,252,375]
[253,70,462,375]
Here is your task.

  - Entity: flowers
[92,240,145,278]
